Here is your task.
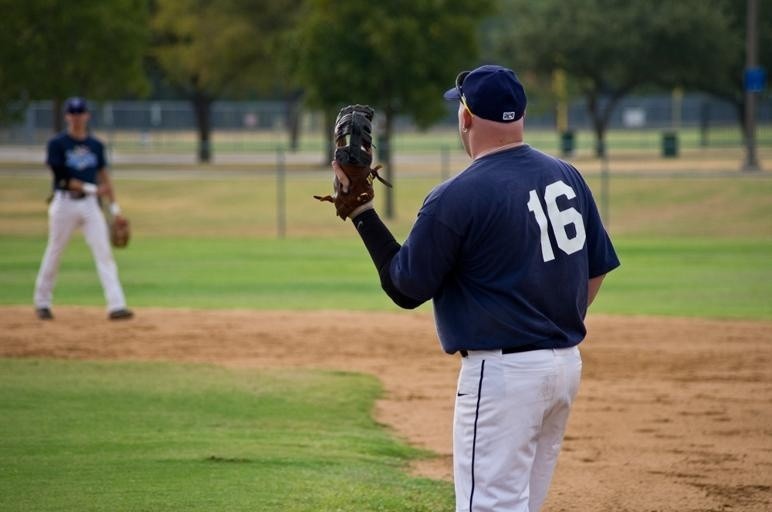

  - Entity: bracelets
[81,182,97,195]
[109,201,121,217]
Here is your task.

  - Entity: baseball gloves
[313,104,391,222]
[112,216,129,246]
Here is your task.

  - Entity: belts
[459,344,532,359]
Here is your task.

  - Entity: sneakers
[109,310,135,322]
[35,309,55,323]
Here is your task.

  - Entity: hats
[445,63,526,125]
[65,95,91,113]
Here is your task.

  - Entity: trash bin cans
[562,133,572,151]
[662,129,677,155]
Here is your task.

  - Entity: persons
[313,64,621,512]
[32,99,134,321]
